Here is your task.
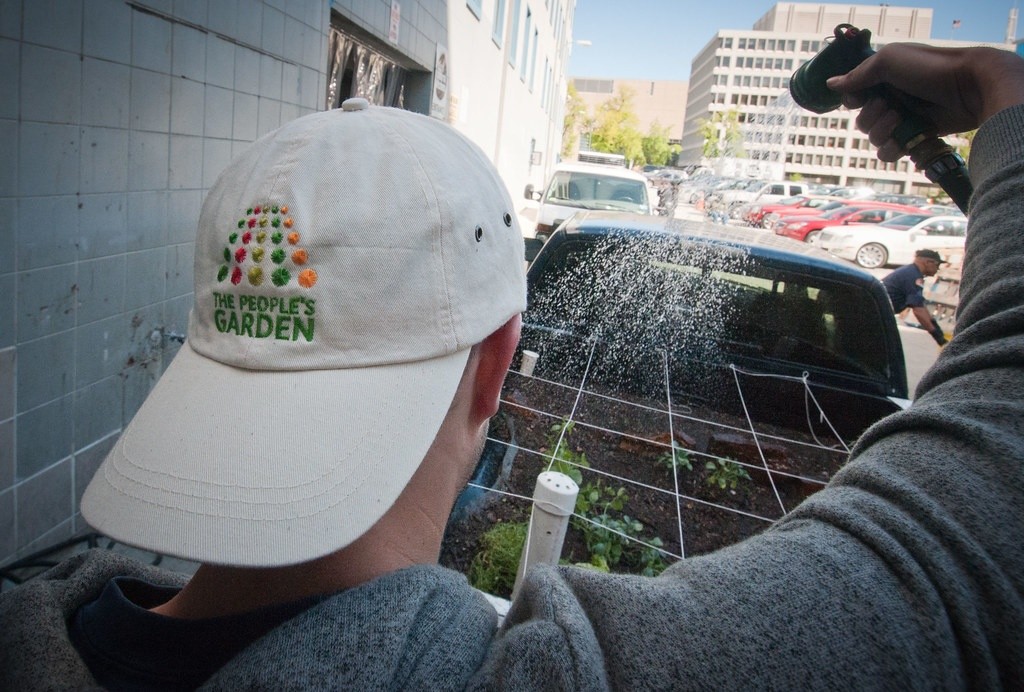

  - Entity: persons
[0,41,1024,692]
[709,201,729,223]
[656,182,678,214]
[881,250,948,345]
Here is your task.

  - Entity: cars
[524,162,653,244]
[815,210,970,268]
[505,208,910,441]
[747,184,931,244]
[640,165,809,219]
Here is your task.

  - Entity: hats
[916,248,948,263]
[81,99,528,566]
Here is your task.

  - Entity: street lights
[542,40,593,188]
